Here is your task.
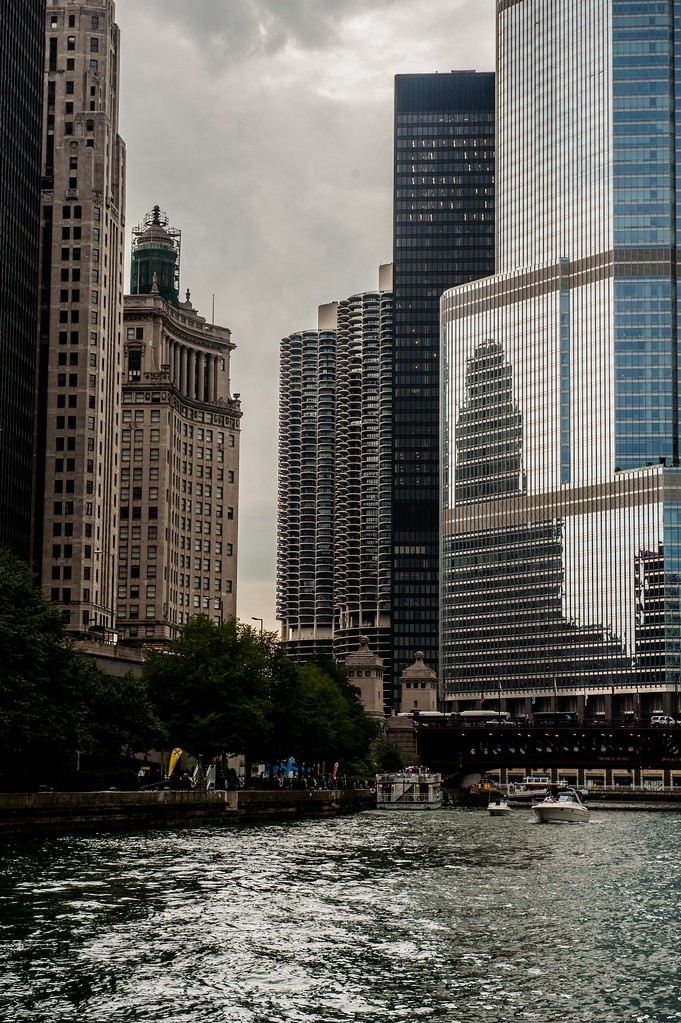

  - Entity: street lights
[205,596,223,625]
[95,549,115,628]
[251,618,262,637]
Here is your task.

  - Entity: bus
[398,710,510,727]
[533,712,578,726]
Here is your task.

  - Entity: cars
[486,718,515,726]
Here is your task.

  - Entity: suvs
[650,716,681,728]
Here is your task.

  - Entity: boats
[501,775,588,807]
[531,785,590,822]
[486,801,512,815]
